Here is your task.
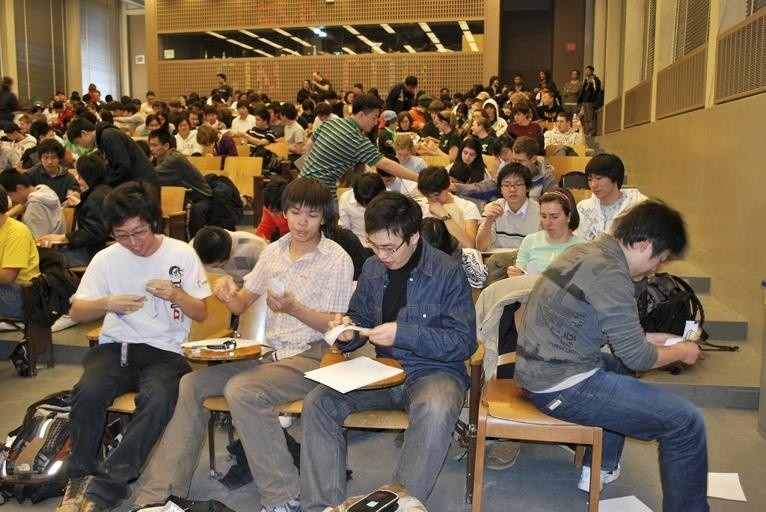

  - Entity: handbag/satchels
[0,389,120,505]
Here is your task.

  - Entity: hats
[378,110,397,129]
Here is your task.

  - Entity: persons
[2,66,710,512]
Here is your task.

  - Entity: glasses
[501,182,525,189]
[109,227,147,241]
[364,236,405,254]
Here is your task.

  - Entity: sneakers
[56,475,108,512]
[486,440,520,470]
[50,313,77,333]
[9,343,30,378]
[0,322,24,331]
[259,495,301,512]
[578,464,620,492]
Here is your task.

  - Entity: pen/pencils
[337,322,362,327]
[182,346,205,349]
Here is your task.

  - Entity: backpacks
[637,272,704,375]
